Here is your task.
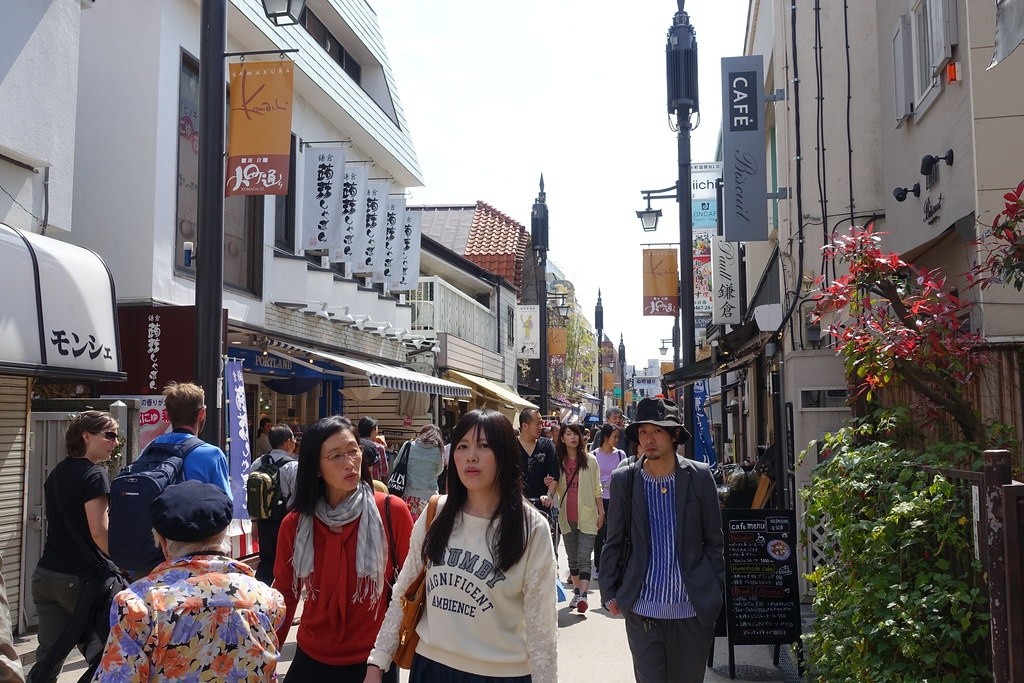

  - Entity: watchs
[549,493,553,498]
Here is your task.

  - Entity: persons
[88,479,286,683]
[24,410,131,683]
[598,398,726,683]
[363,408,561,683]
[250,422,299,588]
[352,406,645,613]
[118,380,234,585]
[271,415,415,683]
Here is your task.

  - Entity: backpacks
[247,453,296,519]
[108,438,204,570]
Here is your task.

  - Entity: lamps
[947,61,962,84]
[921,148,953,174]
[893,183,920,202]
[274,299,439,353]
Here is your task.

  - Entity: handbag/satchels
[554,507,561,534]
[393,494,441,669]
[387,442,411,496]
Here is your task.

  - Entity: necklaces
[570,456,574,463]
[646,461,675,493]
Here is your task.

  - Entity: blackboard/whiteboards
[713,509,802,644]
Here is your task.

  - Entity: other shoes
[567,575,573,583]
[593,572,599,579]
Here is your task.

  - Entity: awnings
[570,387,601,404]
[660,357,751,400]
[447,370,540,413]
[550,396,580,411]
[711,319,772,378]
[0,222,127,383]
[259,336,473,398]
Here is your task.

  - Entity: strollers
[527,498,558,561]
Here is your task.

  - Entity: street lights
[199,0,309,443]
[657,338,682,406]
[637,180,702,456]
[539,291,571,422]
[597,354,615,425]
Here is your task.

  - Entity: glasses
[97,431,120,441]
[321,445,364,463]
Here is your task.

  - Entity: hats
[359,438,380,466]
[150,479,234,542]
[623,396,692,446]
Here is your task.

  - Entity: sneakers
[577,596,588,613]
[569,594,580,607]
